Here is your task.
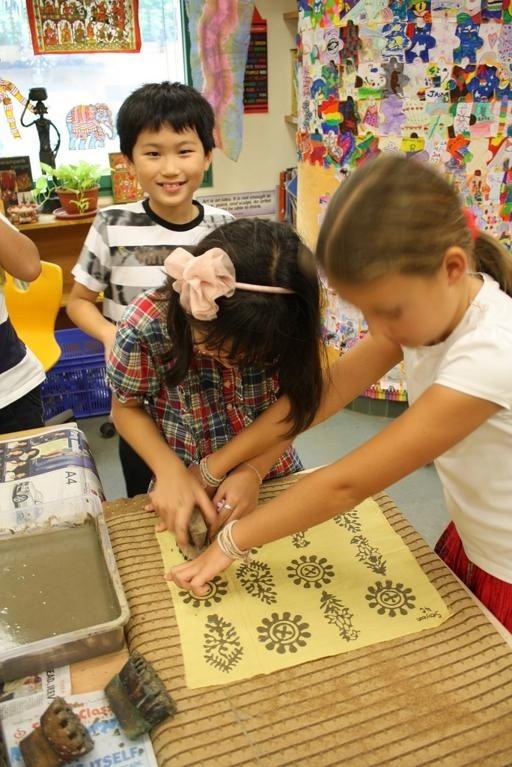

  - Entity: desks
[0,419,161,766]
[92,446,511,767]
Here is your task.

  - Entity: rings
[224,504,232,512]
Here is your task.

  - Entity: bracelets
[199,455,227,488]
[217,521,252,563]
[242,463,262,485]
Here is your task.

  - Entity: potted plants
[32,160,102,222]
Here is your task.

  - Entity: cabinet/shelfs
[283,6,302,129]
[15,204,116,306]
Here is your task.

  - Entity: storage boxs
[2,484,136,684]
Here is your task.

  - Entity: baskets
[38,327,111,422]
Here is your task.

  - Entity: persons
[64,82,237,497]
[145,160,511,642]
[1,214,48,432]
[103,219,330,553]
